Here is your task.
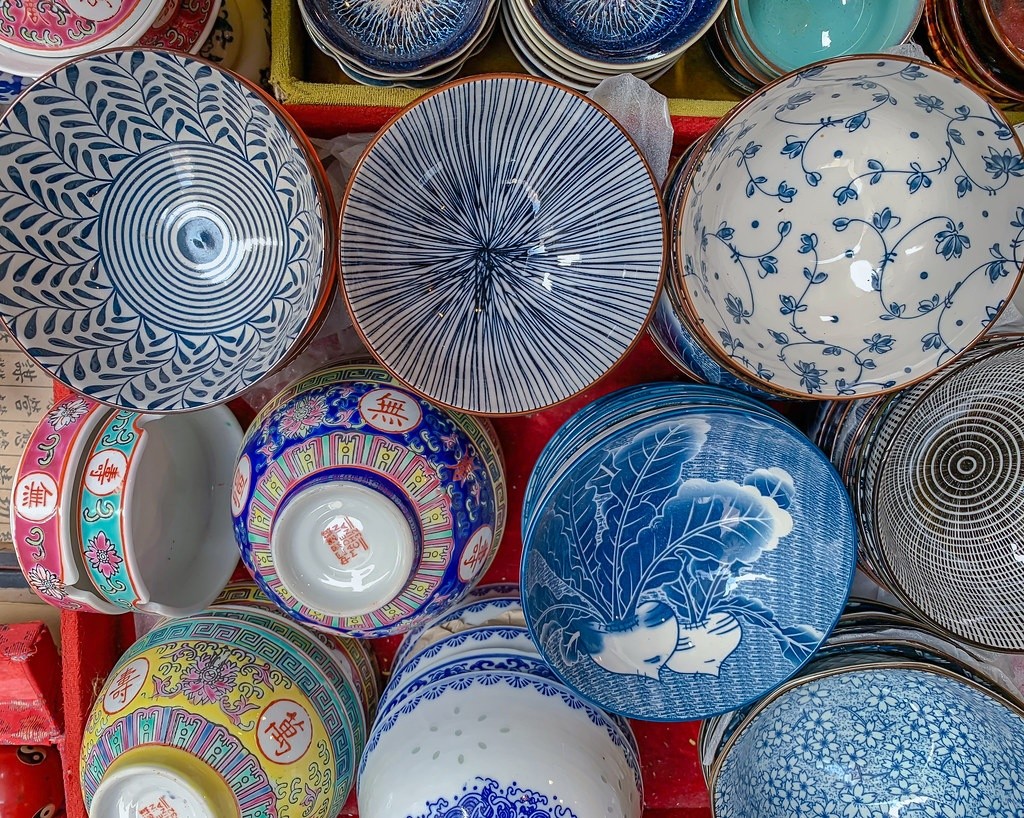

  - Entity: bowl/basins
[1,0,1023,817]
